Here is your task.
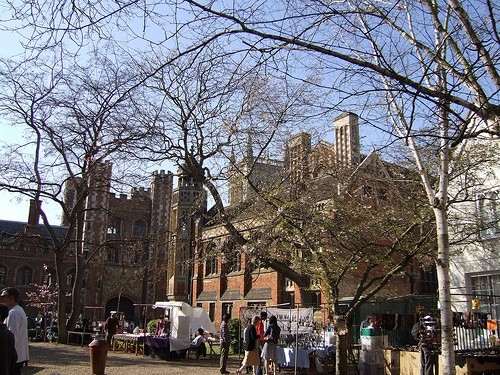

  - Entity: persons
[155,316,170,337]
[105,313,119,350]
[261,316,281,375]
[326,316,335,332]
[219,313,230,375]
[0,304,18,375]
[0,287,29,375]
[179,328,204,359]
[411,315,442,375]
[236,316,262,375]
[256,312,268,375]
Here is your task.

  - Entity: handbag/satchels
[263,325,273,342]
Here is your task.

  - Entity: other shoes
[236,369,241,375]
[221,370,230,375]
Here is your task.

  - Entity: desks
[277,346,327,372]
[145,335,188,362]
[67,331,93,349]
[112,333,152,356]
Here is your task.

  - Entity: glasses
[0,295,10,299]
